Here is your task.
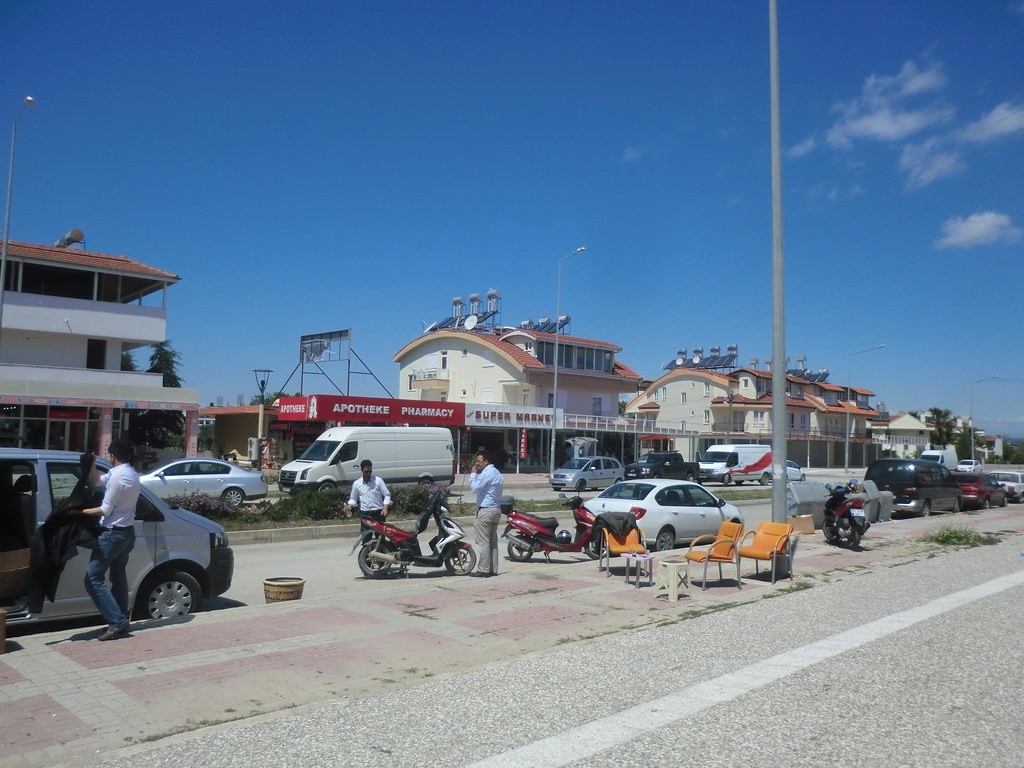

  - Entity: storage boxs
[499,495,515,514]
[786,514,815,533]
[0,546,31,654]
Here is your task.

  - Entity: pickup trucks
[622,450,700,487]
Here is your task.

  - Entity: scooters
[499,481,610,564]
[348,489,477,580]
[822,479,871,552]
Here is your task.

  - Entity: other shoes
[99,620,129,641]
[473,571,489,578]
[490,571,497,576]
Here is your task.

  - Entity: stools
[655,559,691,602]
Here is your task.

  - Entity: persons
[342,459,391,579]
[66,440,141,640]
[468,451,503,577]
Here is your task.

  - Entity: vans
[863,458,964,518]
[276,425,457,496]
[696,444,773,486]
[917,449,959,472]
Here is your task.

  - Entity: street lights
[969,374,1000,461]
[252,368,276,473]
[548,245,589,481]
[843,344,887,474]
[0,96,35,331]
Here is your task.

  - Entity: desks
[620,553,655,589]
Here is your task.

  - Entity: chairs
[737,521,794,586]
[599,520,647,577]
[685,521,743,590]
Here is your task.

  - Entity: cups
[632,551,636,557]
[646,550,650,557]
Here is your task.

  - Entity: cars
[784,459,806,483]
[947,472,1007,510]
[547,455,625,491]
[137,458,269,510]
[0,446,234,627]
[580,477,746,553]
[988,471,1024,503]
[954,459,984,474]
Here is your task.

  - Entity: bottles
[344,502,352,517]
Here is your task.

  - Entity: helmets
[557,530,572,543]
